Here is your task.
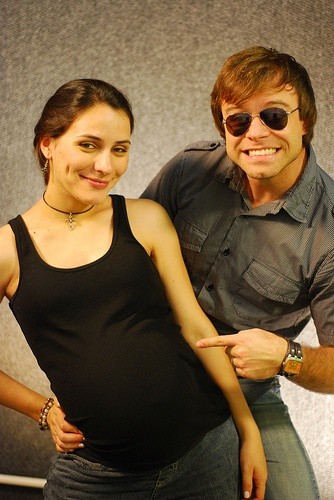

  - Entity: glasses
[221,107,300,137]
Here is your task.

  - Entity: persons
[0,80,269,500]
[138,46,334,500]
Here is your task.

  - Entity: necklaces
[43,188,95,230]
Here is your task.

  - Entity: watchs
[277,339,304,378]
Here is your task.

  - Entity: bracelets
[39,398,54,431]
